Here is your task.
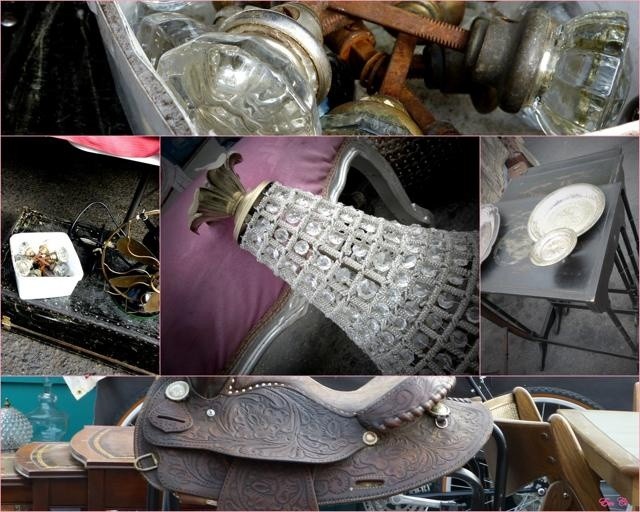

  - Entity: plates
[480,182,605,268]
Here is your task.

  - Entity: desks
[479,148,639,375]
[558,408,638,511]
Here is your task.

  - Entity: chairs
[476,387,609,511]
